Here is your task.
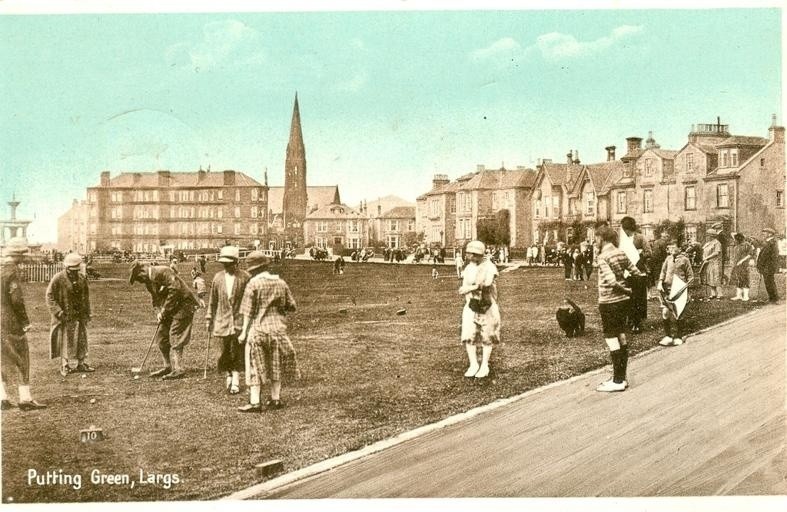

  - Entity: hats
[217,245,240,264]
[245,250,271,271]
[64,253,83,271]
[465,240,486,256]
[704,228,717,237]
[129,260,142,285]
[762,228,776,235]
[711,223,723,230]
[4,237,30,257]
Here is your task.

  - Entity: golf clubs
[197,334,211,381]
[132,324,159,372]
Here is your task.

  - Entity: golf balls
[135,376,139,380]
[83,375,86,379]
[91,400,95,403]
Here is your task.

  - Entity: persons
[236,250,300,413]
[204,246,252,394]
[191,273,207,310]
[457,240,502,378]
[129,258,199,380]
[0,236,47,410]
[593,228,640,394]
[44,252,95,377]
[561,223,786,302]
[620,216,652,334]
[655,237,694,346]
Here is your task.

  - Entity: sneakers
[1,400,18,411]
[18,400,48,410]
[264,399,281,409]
[463,365,490,378]
[76,363,96,372]
[708,295,724,300]
[673,337,683,346]
[162,368,185,380]
[596,377,629,393]
[238,402,262,413]
[149,367,172,377]
[658,335,673,345]
[226,375,240,395]
[61,364,74,374]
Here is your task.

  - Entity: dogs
[555,297,588,339]
[432,268,439,280]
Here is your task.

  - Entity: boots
[742,288,750,302]
[730,287,742,300]
